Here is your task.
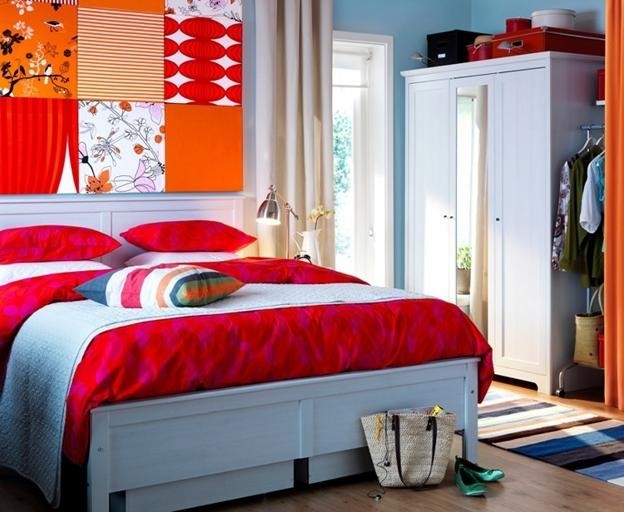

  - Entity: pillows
[0,219,257,307]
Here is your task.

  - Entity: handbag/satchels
[573,311,604,367]
[361,408,457,488]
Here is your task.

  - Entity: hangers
[563,122,605,167]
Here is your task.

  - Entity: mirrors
[454,84,488,342]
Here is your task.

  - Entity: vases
[300,230,322,267]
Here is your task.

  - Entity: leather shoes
[455,455,503,481]
[456,464,488,496]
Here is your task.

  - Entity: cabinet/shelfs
[398,50,607,396]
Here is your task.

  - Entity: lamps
[255,183,299,260]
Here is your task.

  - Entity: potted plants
[455,247,471,295]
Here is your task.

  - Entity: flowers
[305,204,335,230]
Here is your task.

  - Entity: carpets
[455,398,623,488]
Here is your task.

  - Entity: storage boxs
[425,26,605,68]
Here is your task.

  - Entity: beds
[0,195,482,510]
